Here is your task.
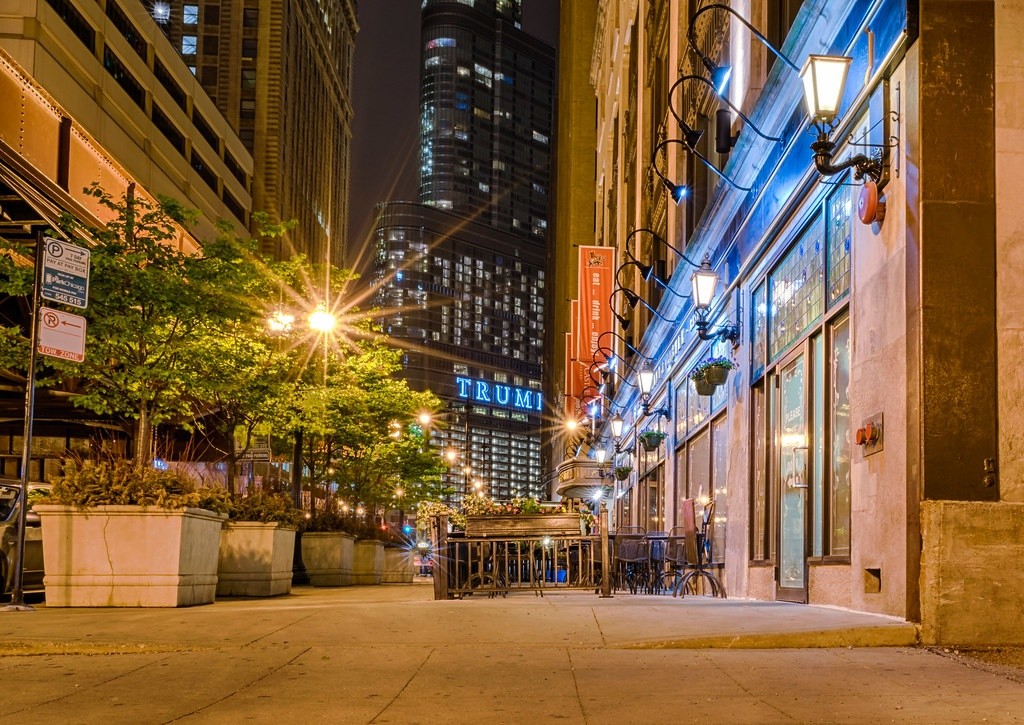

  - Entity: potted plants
[640,435,655,451]
[645,431,665,447]
[613,465,630,480]
[30,458,414,608]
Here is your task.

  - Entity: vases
[466,512,581,536]
[707,365,729,384]
[695,375,717,395]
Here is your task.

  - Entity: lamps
[562,3,892,500]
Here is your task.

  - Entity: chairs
[463,525,728,597]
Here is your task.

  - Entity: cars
[0,477,55,603]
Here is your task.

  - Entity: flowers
[468,497,566,515]
[689,354,740,379]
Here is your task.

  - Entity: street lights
[267,301,339,511]
